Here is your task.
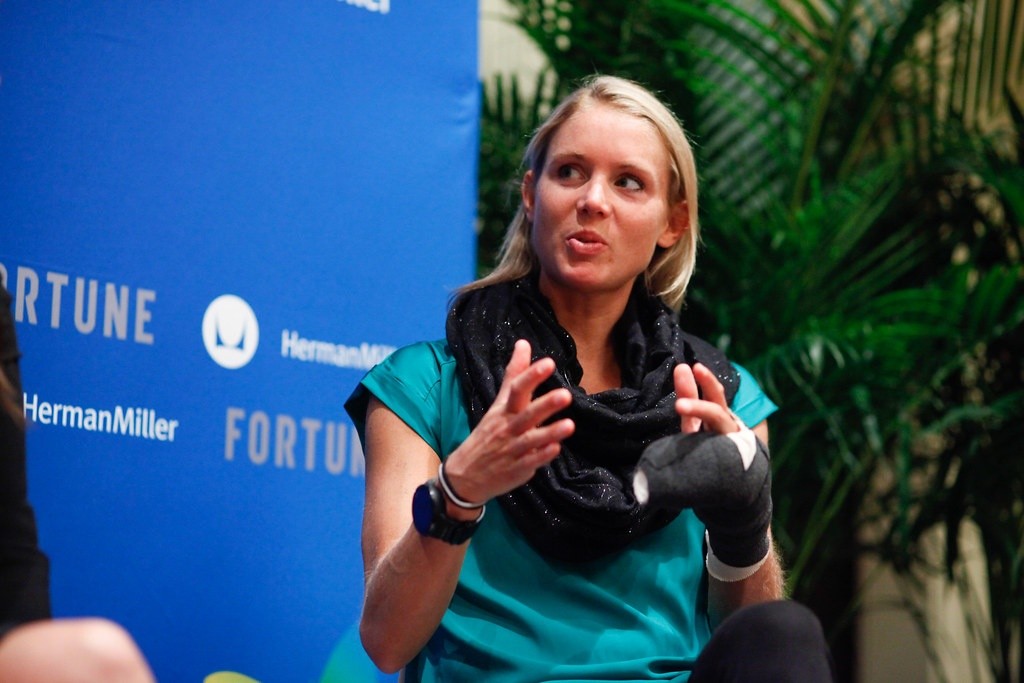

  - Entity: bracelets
[438,457,488,509]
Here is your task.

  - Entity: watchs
[412,476,487,545]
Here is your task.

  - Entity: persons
[342,75,832,683]
